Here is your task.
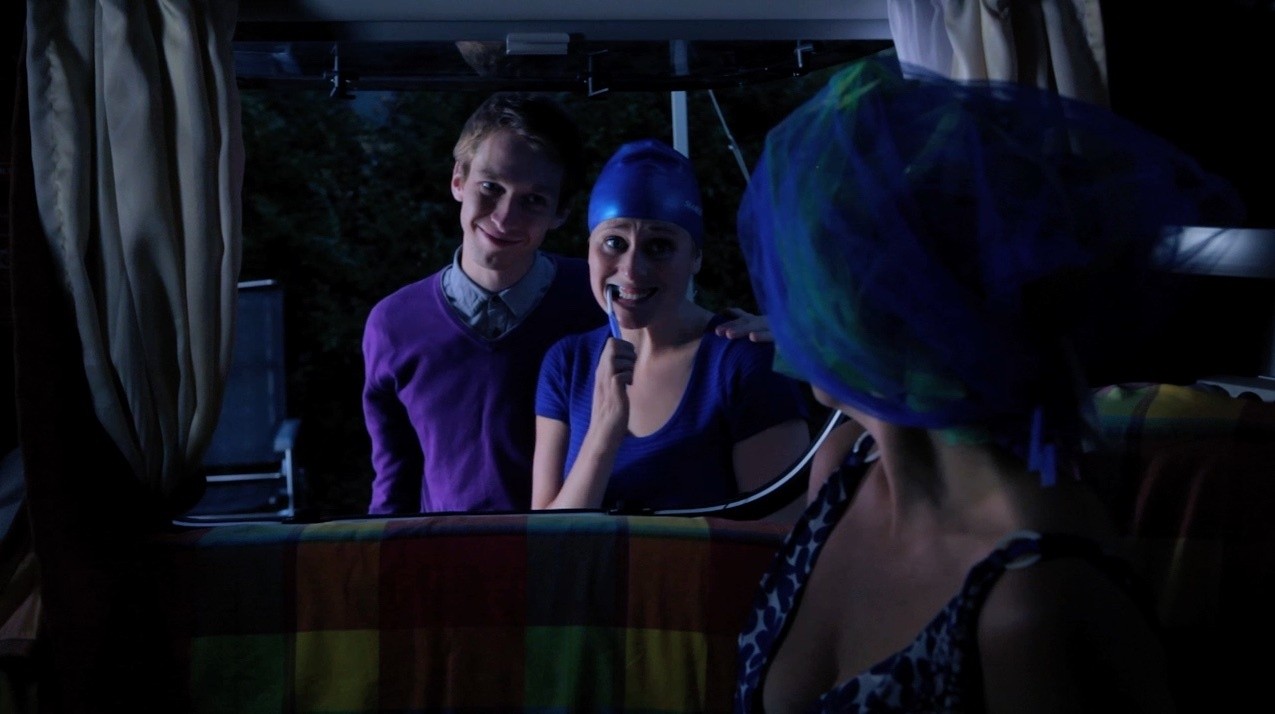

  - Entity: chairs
[166,276,304,527]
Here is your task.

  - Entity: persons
[362,93,774,517]
[534,143,865,513]
[736,57,1184,714]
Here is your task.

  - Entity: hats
[739,53,1068,431]
[586,141,705,244]
